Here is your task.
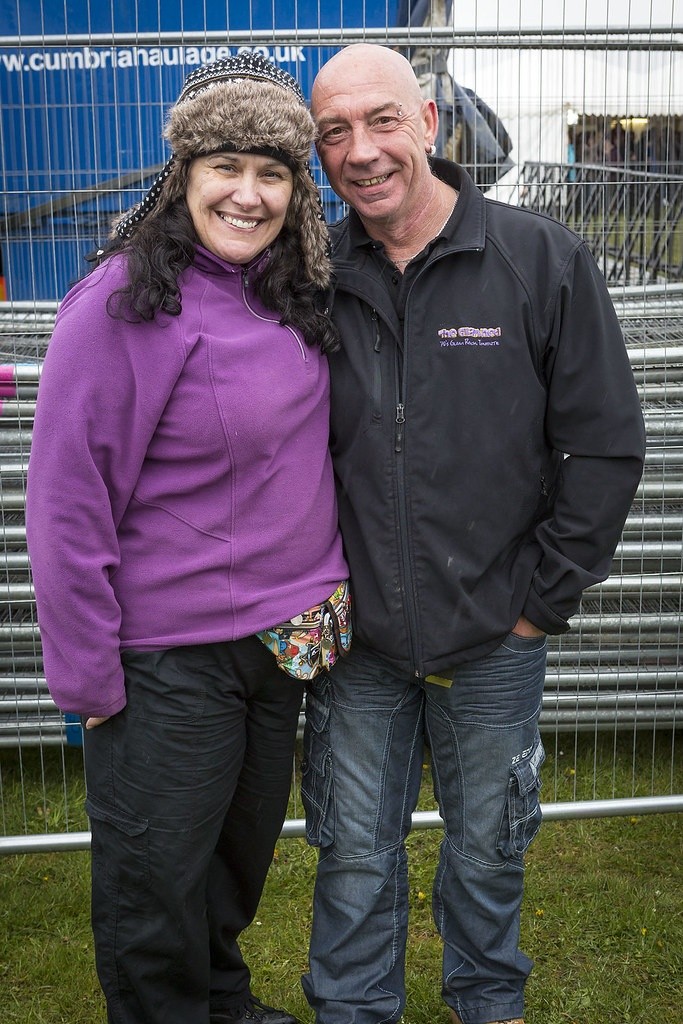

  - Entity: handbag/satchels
[254,579,355,683]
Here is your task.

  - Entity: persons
[301,42,649,1024]
[571,121,683,218]
[25,55,351,1024]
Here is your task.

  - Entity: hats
[109,49,334,291]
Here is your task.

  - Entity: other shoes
[208,993,298,1024]
[448,1006,525,1024]
